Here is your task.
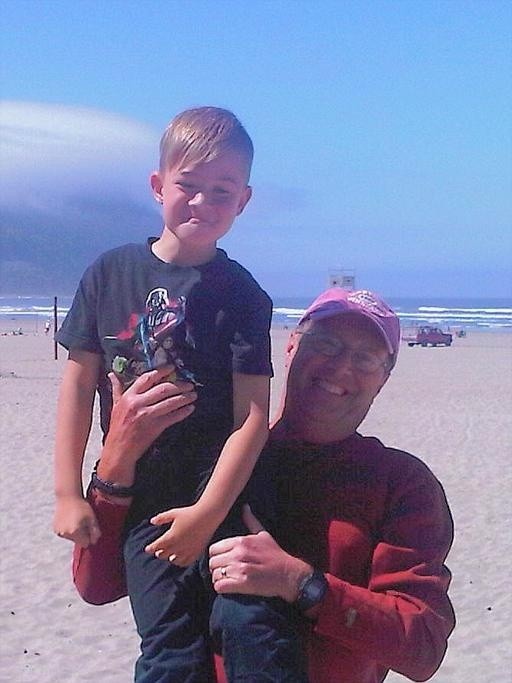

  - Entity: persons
[17,327,23,334]
[49,105,302,682]
[151,335,180,385]
[72,285,454,682]
[45,319,52,335]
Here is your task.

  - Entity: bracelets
[88,473,134,497]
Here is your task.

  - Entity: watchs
[295,565,328,613]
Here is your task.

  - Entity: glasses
[294,331,390,375]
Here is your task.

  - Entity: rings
[221,567,229,578]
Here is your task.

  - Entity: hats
[298,285,402,359]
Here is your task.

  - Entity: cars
[401,324,456,348]
